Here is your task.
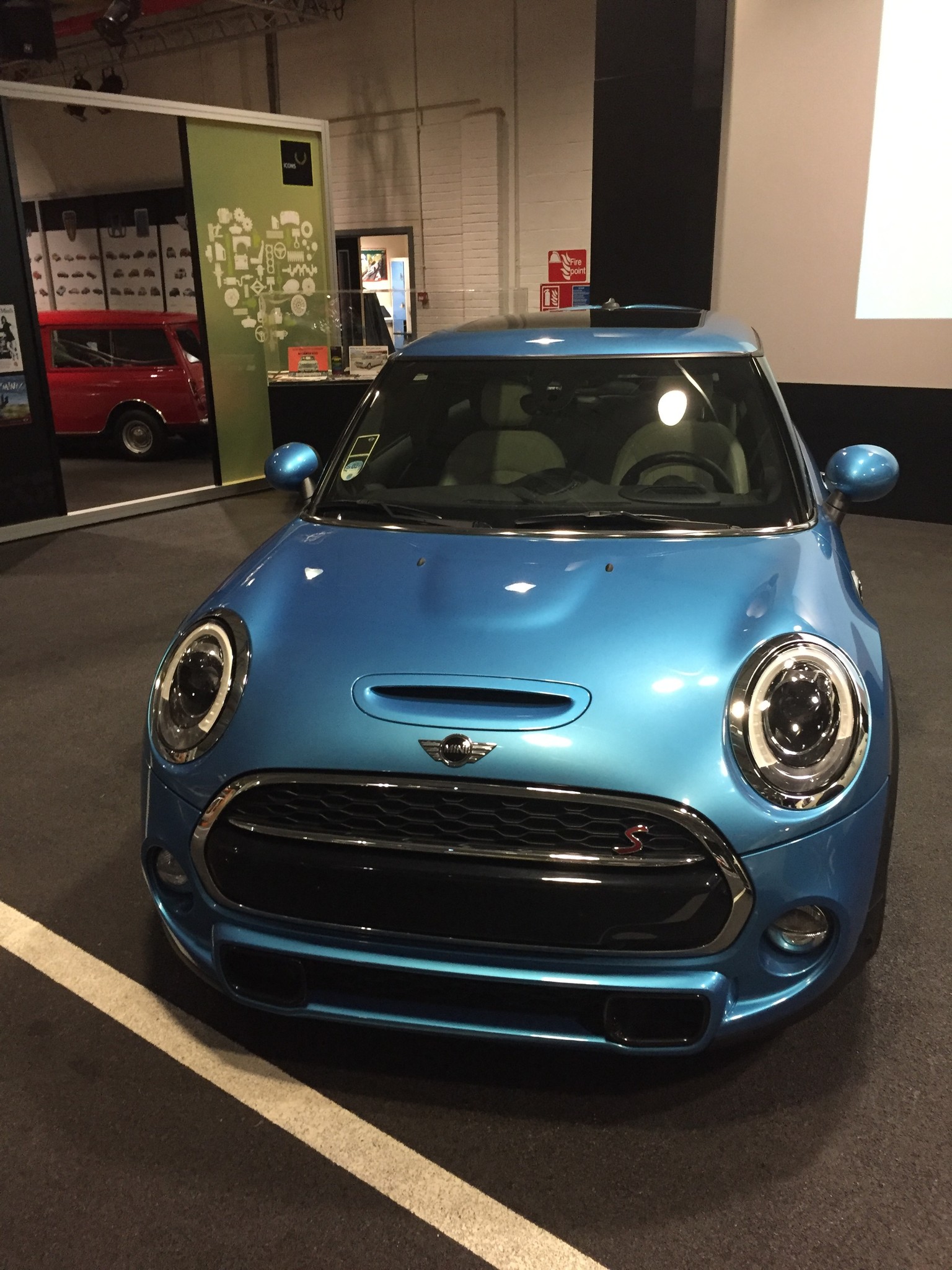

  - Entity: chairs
[440,381,565,487]
[611,375,750,493]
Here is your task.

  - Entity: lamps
[93,0,142,47]
[93,66,123,115]
[63,71,92,122]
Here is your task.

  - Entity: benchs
[532,394,736,483]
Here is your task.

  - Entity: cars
[37,307,213,460]
[136,301,910,1060]
